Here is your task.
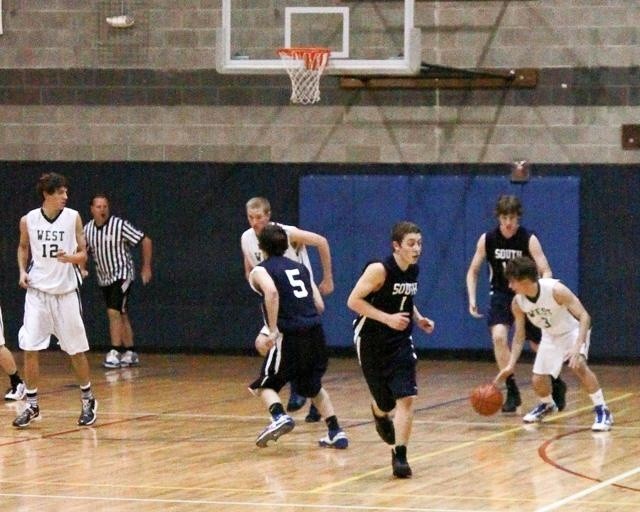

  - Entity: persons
[491,256,615,433]
[75,191,153,368]
[0,308,26,402]
[248,224,347,450]
[13,172,100,427]
[240,194,333,421]
[345,220,435,478]
[466,194,569,415]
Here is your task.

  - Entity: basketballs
[471,383,503,416]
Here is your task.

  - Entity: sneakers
[4,381,42,427]
[318,430,347,449]
[287,382,321,422]
[502,379,567,423]
[256,414,295,447]
[104,349,139,368]
[590,404,615,431]
[76,396,98,426]
[371,401,411,476]
